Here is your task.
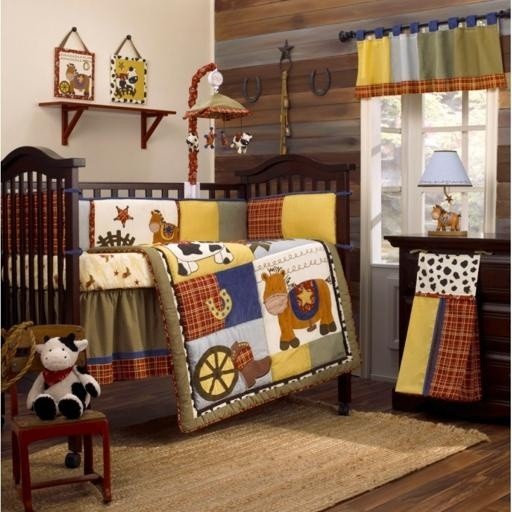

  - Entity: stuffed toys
[26,333,102,419]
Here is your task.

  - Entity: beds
[1,145,357,469]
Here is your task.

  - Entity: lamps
[418,150,474,237]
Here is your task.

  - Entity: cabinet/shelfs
[386,235,511,421]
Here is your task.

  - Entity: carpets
[1,403,489,512]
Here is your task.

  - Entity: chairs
[1,324,112,512]
